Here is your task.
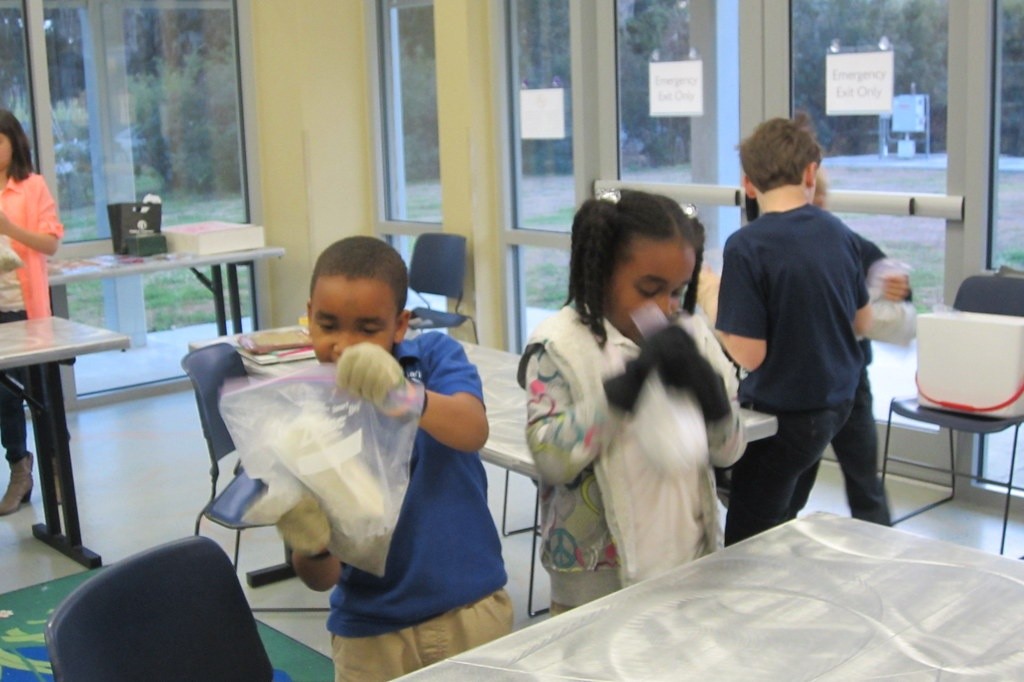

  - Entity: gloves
[667,351,733,423]
[603,324,696,414]
[275,490,336,558]
[334,342,431,422]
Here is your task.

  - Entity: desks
[388,511,1024,682]
[458,340,777,618]
[187,324,551,617]
[0,316,130,571]
[49,248,287,337]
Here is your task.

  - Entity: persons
[715,112,914,546]
[275,236,513,682]
[0,110,64,515]
[517,187,748,619]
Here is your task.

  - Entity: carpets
[0,564,335,682]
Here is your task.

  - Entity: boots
[0,452,34,517]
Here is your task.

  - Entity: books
[237,331,316,365]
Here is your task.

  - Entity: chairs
[44,535,274,682]
[406,233,479,342]
[182,342,277,574]
[880,275,1024,559]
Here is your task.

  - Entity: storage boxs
[164,221,265,255]
[128,233,167,256]
